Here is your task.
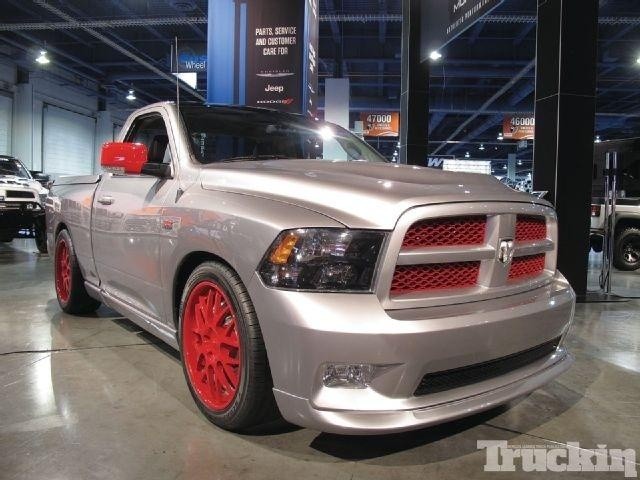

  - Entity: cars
[590,136,639,270]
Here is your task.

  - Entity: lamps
[34,40,51,66]
[125,82,139,101]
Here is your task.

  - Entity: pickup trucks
[43,99,577,438]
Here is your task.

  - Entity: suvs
[1,155,48,253]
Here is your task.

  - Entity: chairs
[251,136,303,158]
[148,135,194,164]
